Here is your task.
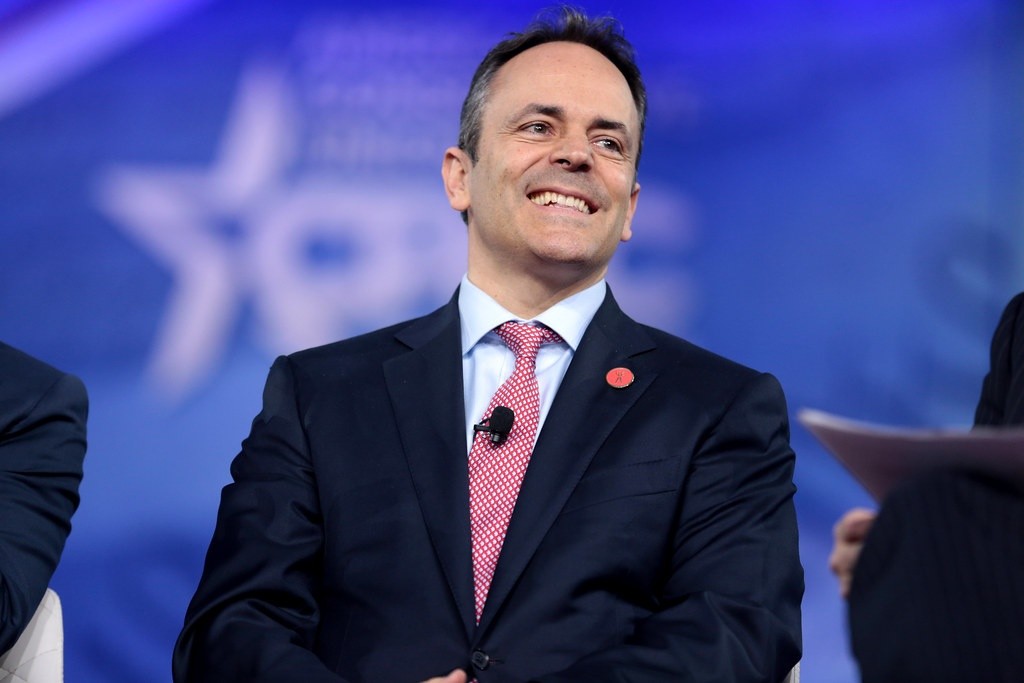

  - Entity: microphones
[488,406,515,446]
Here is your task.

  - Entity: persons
[0,342,87,656]
[828,290,1024,683]
[171,7,804,683]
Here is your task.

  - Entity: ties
[468,320,567,626]
